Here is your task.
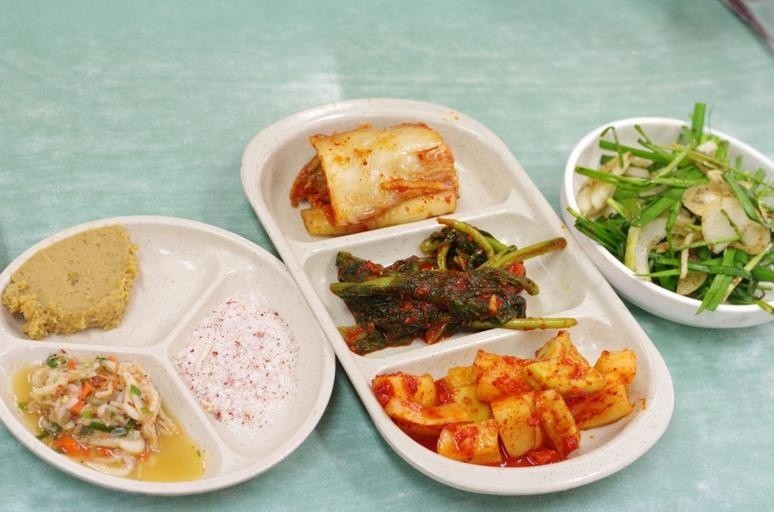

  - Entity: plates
[0,215,336,497]
[240,98,675,497]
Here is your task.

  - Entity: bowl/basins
[559,116,773,330]
